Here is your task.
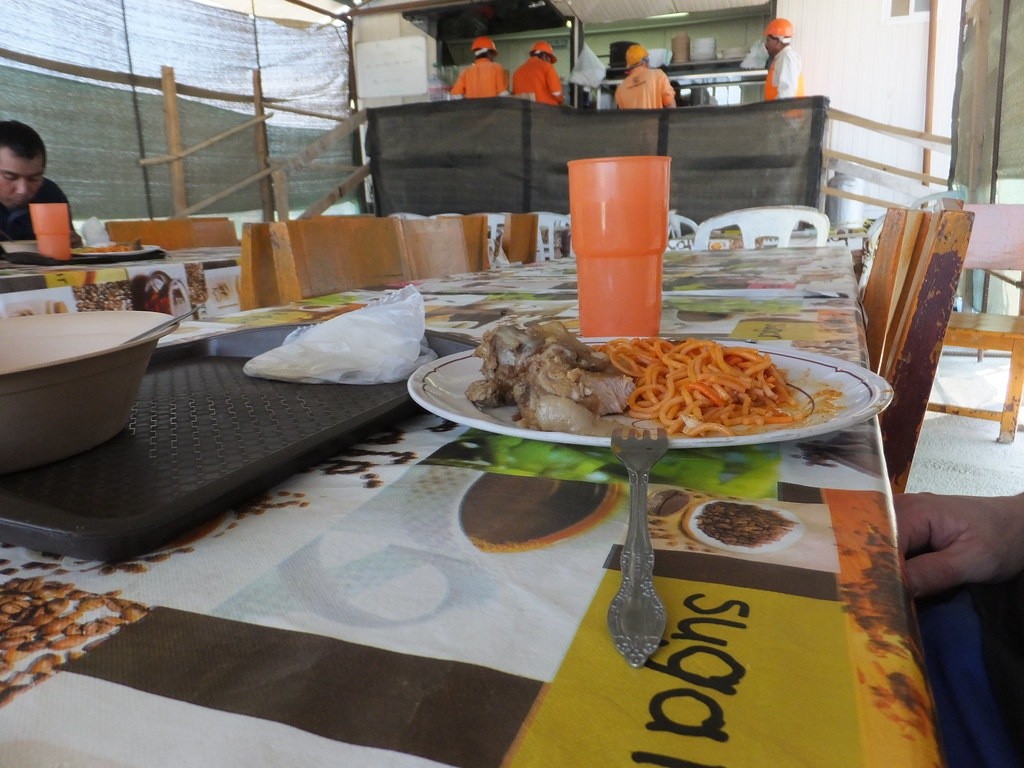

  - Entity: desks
[665,229,834,251]
[0,244,942,768]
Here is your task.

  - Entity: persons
[670,80,688,107]
[0,119,85,248]
[893,491,1024,768]
[614,45,676,108]
[448,35,511,97]
[764,17,805,131]
[513,40,565,105]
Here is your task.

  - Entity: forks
[608,424,672,668]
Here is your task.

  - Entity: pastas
[84,245,129,252]
[589,337,798,434]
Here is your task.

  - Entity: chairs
[239,211,580,311]
[921,200,1024,445]
[694,204,831,251]
[104,220,239,251]
[877,197,976,493]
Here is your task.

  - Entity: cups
[28,203,72,261]
[567,155,672,340]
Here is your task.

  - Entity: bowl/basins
[0,239,40,255]
[0,309,180,470]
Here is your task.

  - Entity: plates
[408,333,895,451]
[691,38,718,60]
[720,48,746,59]
[74,244,161,255]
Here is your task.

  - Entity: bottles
[671,31,690,62]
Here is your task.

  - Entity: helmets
[471,36,498,56]
[762,18,794,36]
[626,44,648,66]
[529,40,557,63]
